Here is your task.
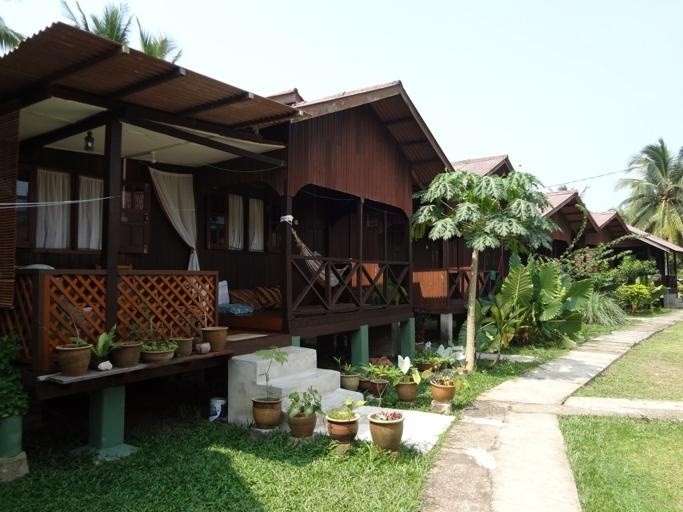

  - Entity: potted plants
[250,346,287,428]
[395,356,420,402]
[287,384,322,437]
[56,326,228,377]
[424,342,469,402]
[368,406,407,450]
[325,398,366,443]
[335,356,395,396]
